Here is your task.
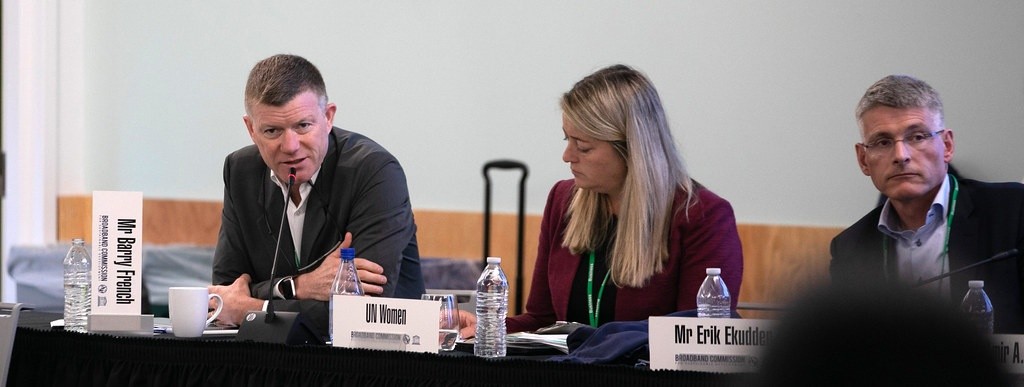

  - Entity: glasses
[861,129,944,155]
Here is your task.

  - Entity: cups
[421,294,460,352]
[168,286,224,337]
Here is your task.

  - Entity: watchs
[282,276,298,300]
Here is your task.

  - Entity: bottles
[697,268,731,318]
[328,248,365,346]
[473,257,509,357]
[63,238,92,332]
[962,280,994,333]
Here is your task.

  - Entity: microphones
[908,248,1020,289]
[235,168,328,346]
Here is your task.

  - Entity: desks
[5,310,760,387]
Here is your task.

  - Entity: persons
[437,65,743,338]
[829,75,1024,335]
[762,279,1012,387]
[206,55,425,328]
